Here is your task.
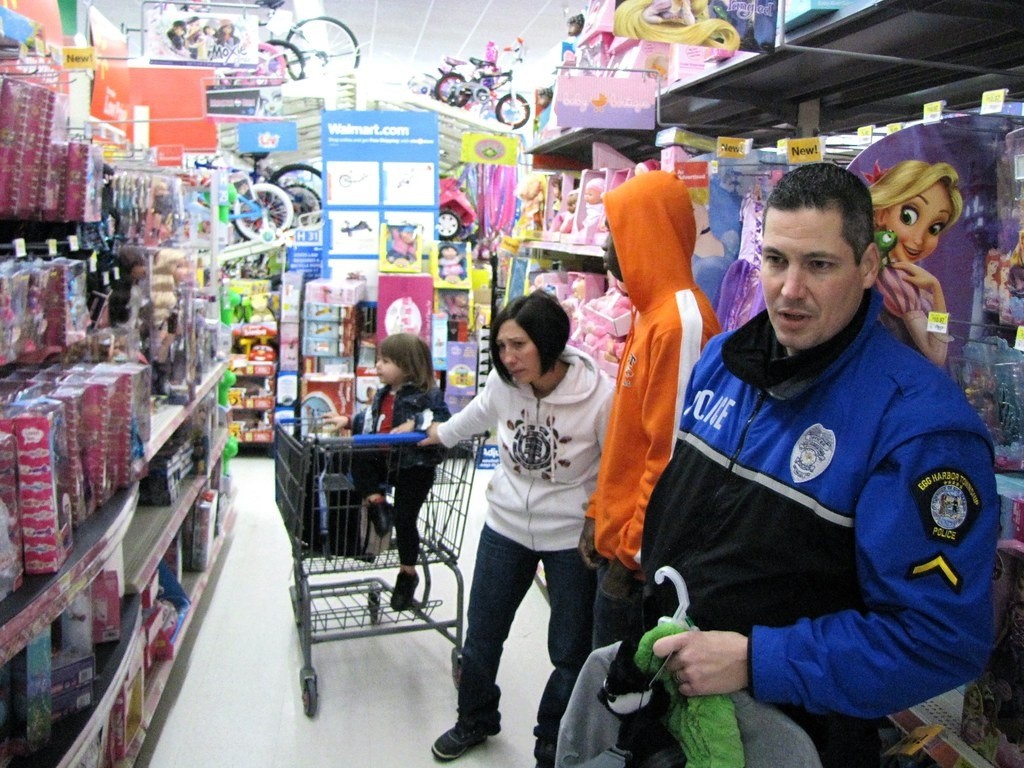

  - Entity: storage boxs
[501,167,632,336]
[0,76,218,768]
[271,223,476,432]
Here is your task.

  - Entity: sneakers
[432,721,487,759]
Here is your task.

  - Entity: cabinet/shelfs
[220,226,291,453]
[484,4,1024,768]
[1,0,234,768]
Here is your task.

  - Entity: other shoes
[391,570,419,611]
[367,498,395,536]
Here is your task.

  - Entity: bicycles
[405,36,532,131]
[192,151,324,242]
[123,0,362,90]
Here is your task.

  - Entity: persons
[640,163,1000,768]
[170,16,241,62]
[102,338,121,363]
[415,288,616,767]
[983,229,1024,355]
[149,181,174,244]
[108,247,148,333]
[518,174,546,229]
[446,292,470,341]
[578,169,724,652]
[582,177,605,231]
[560,188,579,233]
[547,171,563,230]
[438,247,463,281]
[151,247,187,395]
[101,164,124,240]
[866,159,963,368]
[13,222,125,294]
[387,226,417,263]
[560,277,586,342]
[317,332,452,611]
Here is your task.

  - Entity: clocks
[435,210,462,240]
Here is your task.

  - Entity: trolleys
[272,412,492,720]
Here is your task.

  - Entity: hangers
[654,564,700,633]
